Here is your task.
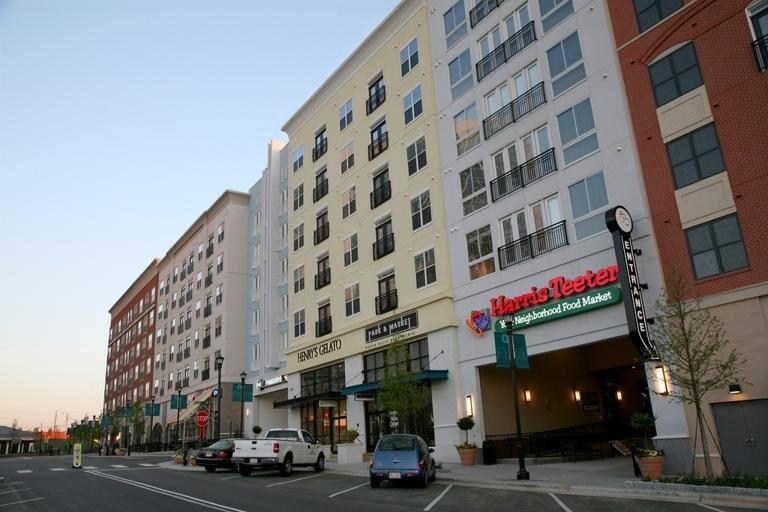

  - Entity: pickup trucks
[230,428,325,476]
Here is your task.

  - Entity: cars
[370,434,436,487]
[196,437,245,473]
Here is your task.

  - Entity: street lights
[502,311,526,472]
[239,369,247,438]
[149,396,155,449]
[216,355,223,440]
[176,386,182,445]
[79,400,130,452]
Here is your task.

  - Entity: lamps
[575,390,581,402]
[466,395,474,417]
[616,390,623,401]
[525,389,532,402]
[729,384,741,394]
[655,366,668,394]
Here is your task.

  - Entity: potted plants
[455,415,478,465]
[631,412,667,481]
[339,429,360,442]
